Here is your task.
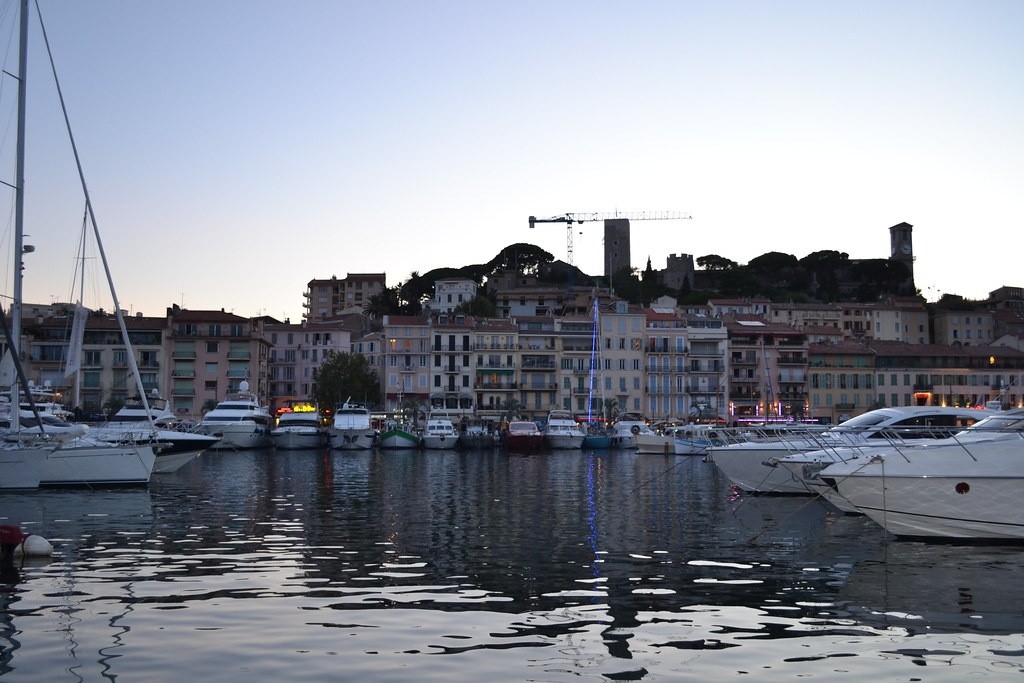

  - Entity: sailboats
[0,2,173,487]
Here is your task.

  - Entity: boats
[805,414,1023,539]
[462,427,501,446]
[102,388,181,430]
[667,426,714,455]
[378,391,420,448]
[506,422,543,447]
[272,401,329,448]
[201,381,273,447]
[422,410,460,450]
[698,404,1004,493]
[330,396,376,449]
[634,433,674,454]
[756,407,1024,515]
[587,421,655,450]
[543,410,587,450]
[85,425,222,474]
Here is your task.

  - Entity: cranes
[528,212,695,265]
